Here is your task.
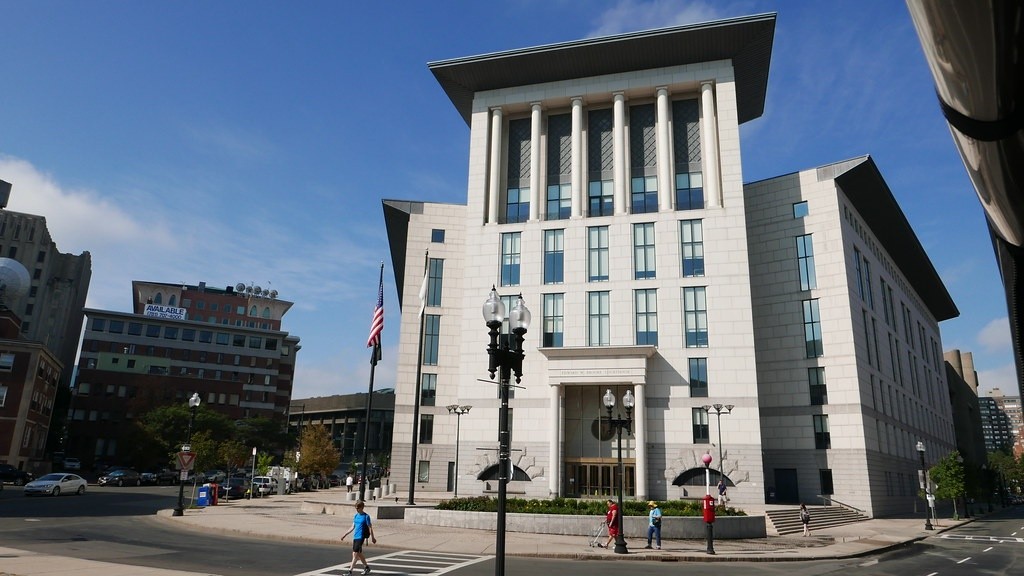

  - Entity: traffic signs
[181,444,191,452]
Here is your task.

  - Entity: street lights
[603,389,637,553]
[447,404,472,498]
[956,453,996,518]
[283,404,306,494]
[703,402,736,483]
[172,393,201,517]
[482,283,532,576]
[915,440,936,532]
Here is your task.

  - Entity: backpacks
[355,513,370,546]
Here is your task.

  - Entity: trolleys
[589,522,605,547]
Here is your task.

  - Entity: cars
[218,478,257,499]
[187,471,205,485]
[204,470,225,483]
[251,476,278,495]
[326,464,386,486]
[62,457,80,473]
[24,472,87,497]
[97,470,141,487]
[0,463,37,486]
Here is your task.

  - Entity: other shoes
[601,542,608,548]
[655,547,661,550]
[360,567,370,575]
[612,545,616,549]
[803,535,806,537]
[342,570,352,576]
[808,533,811,536]
[645,545,652,549]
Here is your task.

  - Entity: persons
[645,501,662,549]
[799,503,811,538]
[603,500,627,548]
[716,480,727,508]
[346,474,353,493]
[340,502,377,576]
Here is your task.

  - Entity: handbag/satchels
[804,515,810,521]
[654,518,661,527]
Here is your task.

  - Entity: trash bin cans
[197,482,219,506]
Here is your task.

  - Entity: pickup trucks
[139,469,180,486]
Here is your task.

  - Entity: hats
[647,501,657,508]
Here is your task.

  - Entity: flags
[365,279,384,366]
